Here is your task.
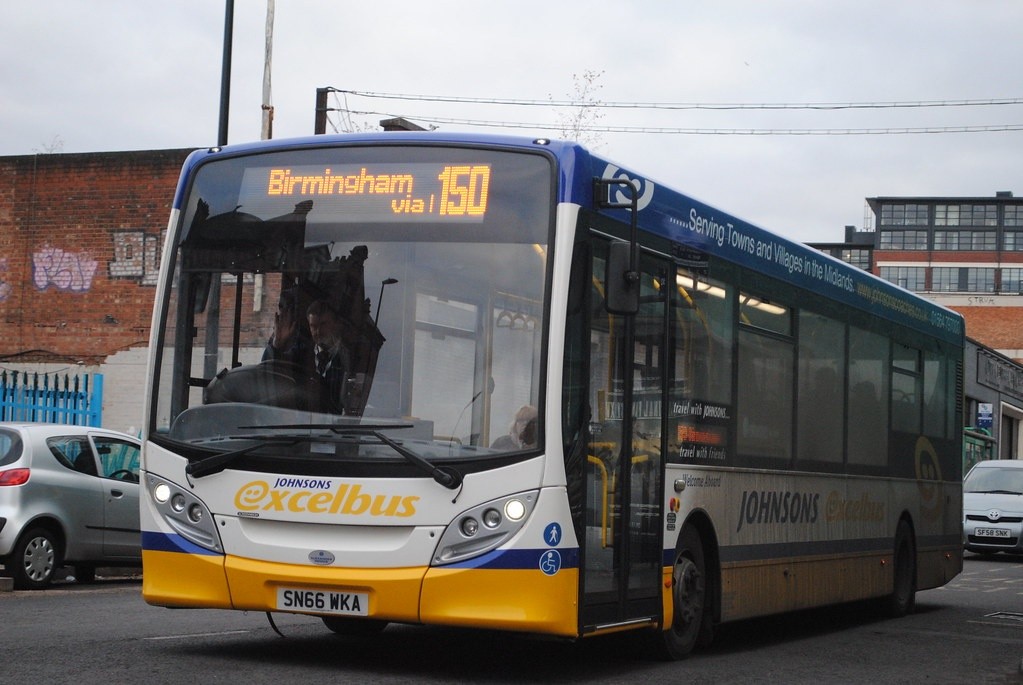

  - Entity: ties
[317,351,330,375]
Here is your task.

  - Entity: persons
[487,406,538,451]
[261,299,358,411]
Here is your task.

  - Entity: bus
[138,131,965,663]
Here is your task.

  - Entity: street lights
[356,277,399,416]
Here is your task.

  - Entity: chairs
[890,387,946,440]
[73,451,98,476]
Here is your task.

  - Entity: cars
[0,421,142,590]
[963,459,1023,556]
